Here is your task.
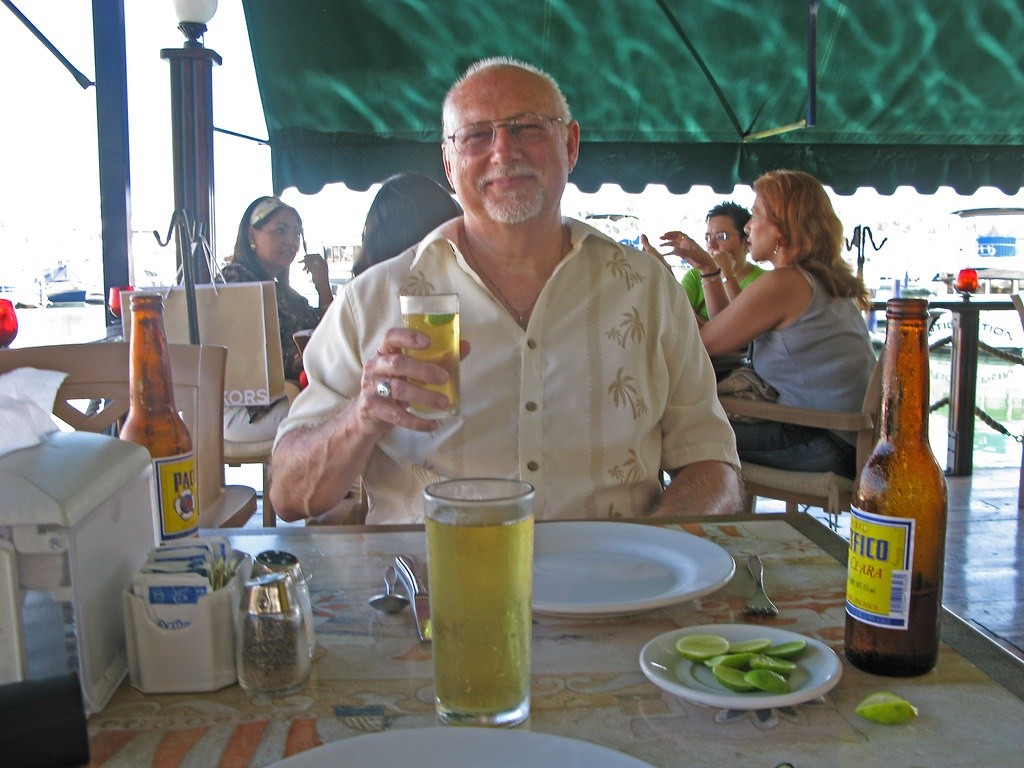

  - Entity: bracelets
[721,274,738,283]
[698,275,720,288]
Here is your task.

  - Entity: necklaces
[459,220,565,332]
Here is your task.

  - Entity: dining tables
[84,510,1023,768]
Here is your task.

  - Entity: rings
[374,374,393,399]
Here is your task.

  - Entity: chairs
[1,278,288,529]
[714,344,887,512]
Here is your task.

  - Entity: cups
[398,292,461,418]
[423,477,536,730]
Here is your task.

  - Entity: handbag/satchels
[717,367,779,425]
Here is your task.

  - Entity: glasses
[705,230,741,240]
[445,110,568,157]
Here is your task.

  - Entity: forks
[742,551,782,617]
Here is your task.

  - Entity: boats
[927,268,1023,350]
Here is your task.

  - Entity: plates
[638,622,844,711]
[530,519,736,621]
[267,727,656,768]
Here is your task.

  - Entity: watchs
[316,304,329,311]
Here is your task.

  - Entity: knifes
[395,554,432,641]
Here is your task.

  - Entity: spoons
[367,566,412,615]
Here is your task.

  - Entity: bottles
[234,548,316,693]
[846,296,950,678]
[117,294,201,544]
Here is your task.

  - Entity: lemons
[676,633,806,692]
[424,313,455,326]
[855,691,919,724]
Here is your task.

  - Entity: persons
[681,201,769,318]
[638,169,878,481]
[208,195,336,443]
[268,56,742,526]
